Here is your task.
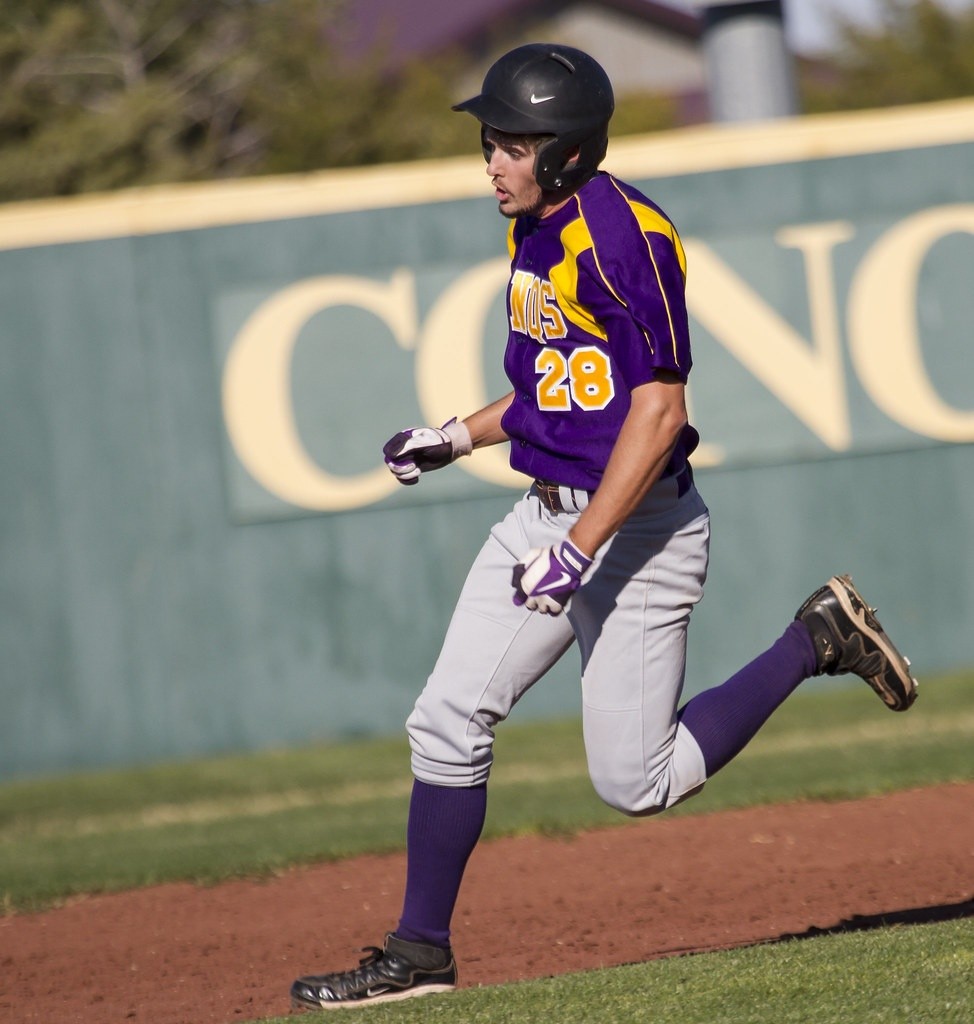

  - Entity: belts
[532,463,695,514]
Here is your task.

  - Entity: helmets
[452,42,615,192]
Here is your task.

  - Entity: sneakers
[794,574,920,712]
[290,931,459,1012]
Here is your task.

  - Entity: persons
[292,45,918,1010]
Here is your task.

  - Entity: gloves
[511,535,594,616]
[384,417,473,484]
